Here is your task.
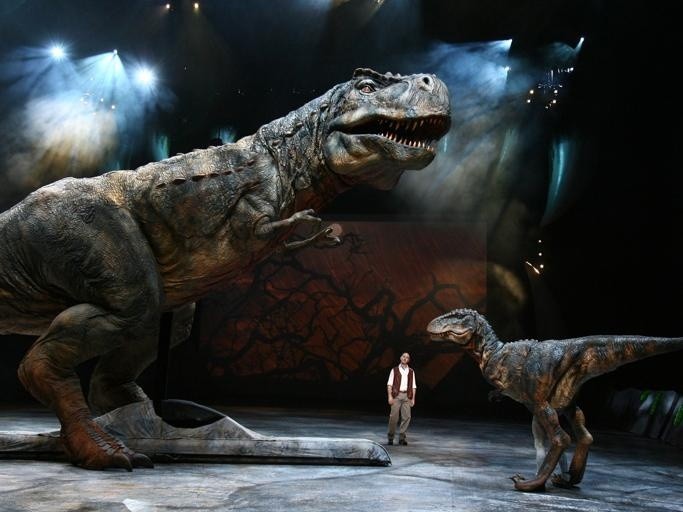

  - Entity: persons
[385,351,417,446]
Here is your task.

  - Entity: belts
[399,391,407,393]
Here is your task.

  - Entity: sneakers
[399,440,407,445]
[388,439,393,445]
